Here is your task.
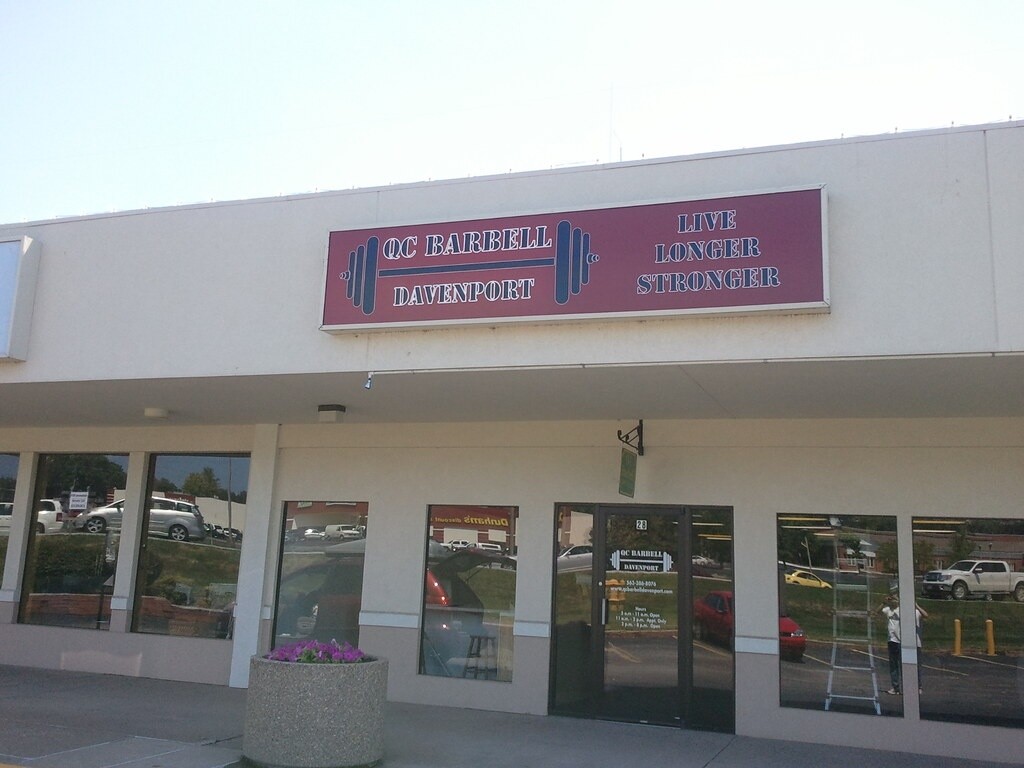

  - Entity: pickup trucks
[920,559,1024,605]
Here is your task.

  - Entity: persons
[915,602,928,695]
[877,592,903,696]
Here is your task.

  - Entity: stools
[463,636,497,680]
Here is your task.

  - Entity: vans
[325,524,361,542]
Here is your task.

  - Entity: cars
[36,499,64,534]
[213,546,508,675]
[472,542,503,560]
[691,590,807,663]
[0,502,14,537]
[555,544,595,573]
[305,528,325,541]
[785,569,833,589]
[204,522,242,541]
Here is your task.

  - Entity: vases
[242,653,388,768]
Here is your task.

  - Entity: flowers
[269,639,365,663]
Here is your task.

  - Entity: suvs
[438,540,471,551]
[71,495,206,543]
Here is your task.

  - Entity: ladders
[822,525,882,716]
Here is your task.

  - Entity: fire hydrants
[605,578,625,611]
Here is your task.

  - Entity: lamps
[145,408,169,420]
[317,404,346,424]
